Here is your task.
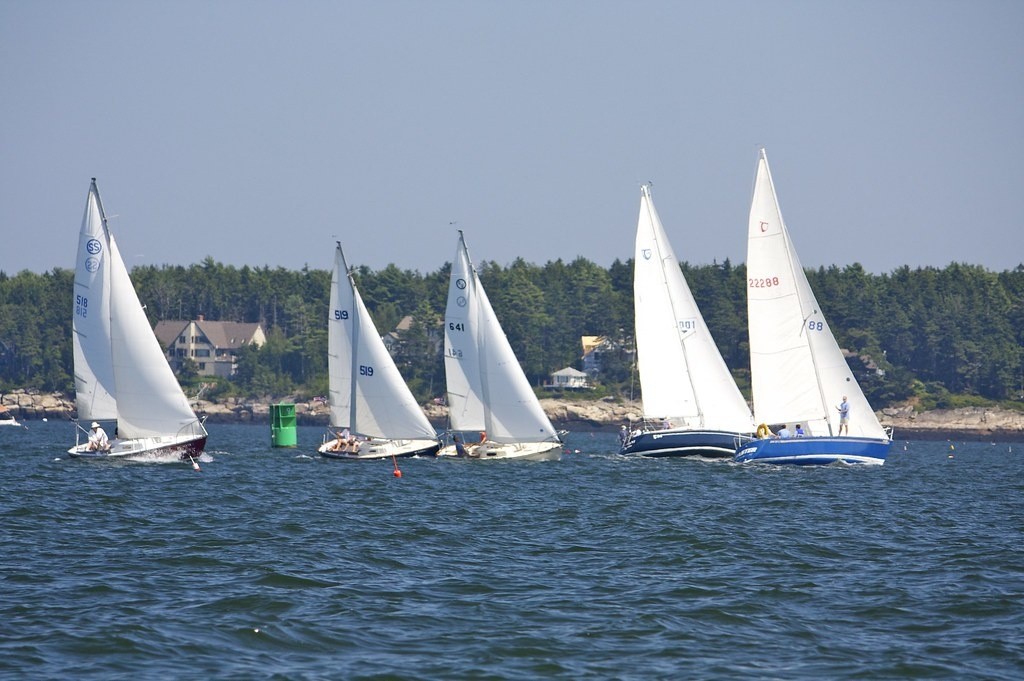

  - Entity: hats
[91,422,100,429]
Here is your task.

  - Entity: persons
[480,431,487,444]
[330,428,368,452]
[86,421,112,451]
[619,424,629,447]
[794,424,805,438]
[628,424,642,442]
[777,425,791,439]
[835,396,850,437]
[453,435,467,458]
[660,418,670,430]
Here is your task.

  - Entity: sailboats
[67,176,209,473]
[731,148,894,467]
[618,180,759,459]
[317,240,446,461]
[436,229,569,463]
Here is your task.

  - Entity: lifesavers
[755,422,769,438]
